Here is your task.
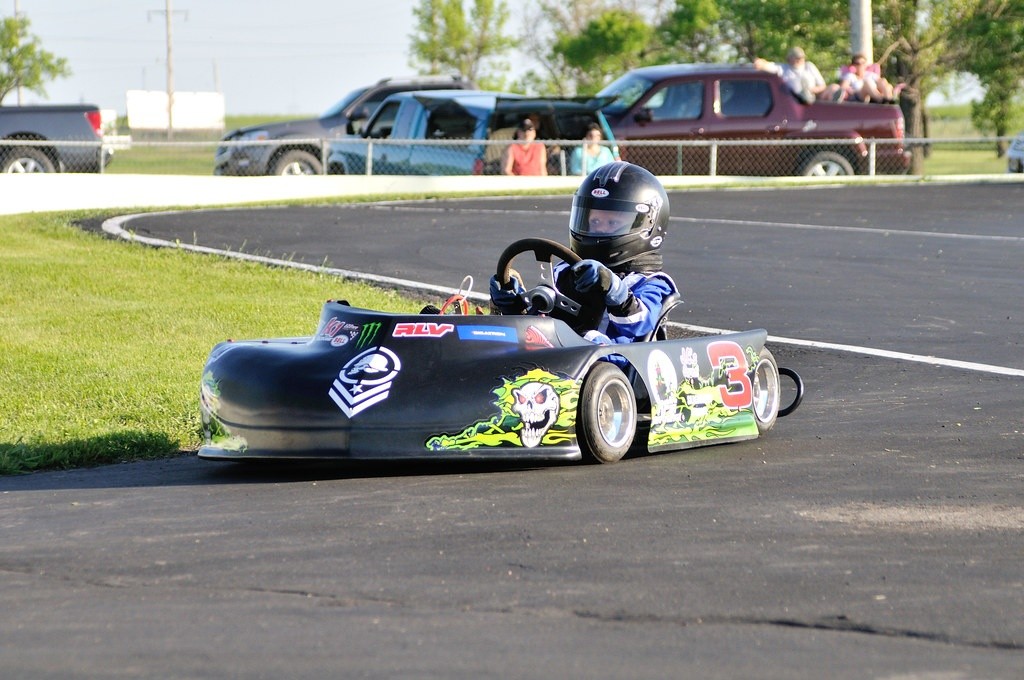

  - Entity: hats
[518,118,536,132]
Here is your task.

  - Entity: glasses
[521,127,535,132]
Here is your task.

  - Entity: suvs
[325,91,621,178]
[211,74,485,176]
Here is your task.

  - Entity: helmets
[567,160,671,269]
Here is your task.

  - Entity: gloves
[570,260,632,308]
[489,272,530,316]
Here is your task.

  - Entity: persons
[490,162,680,344]
[571,123,614,176]
[751,46,839,102]
[843,55,895,105]
[501,118,548,175]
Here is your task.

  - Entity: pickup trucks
[591,63,912,177]
[0,104,112,175]
